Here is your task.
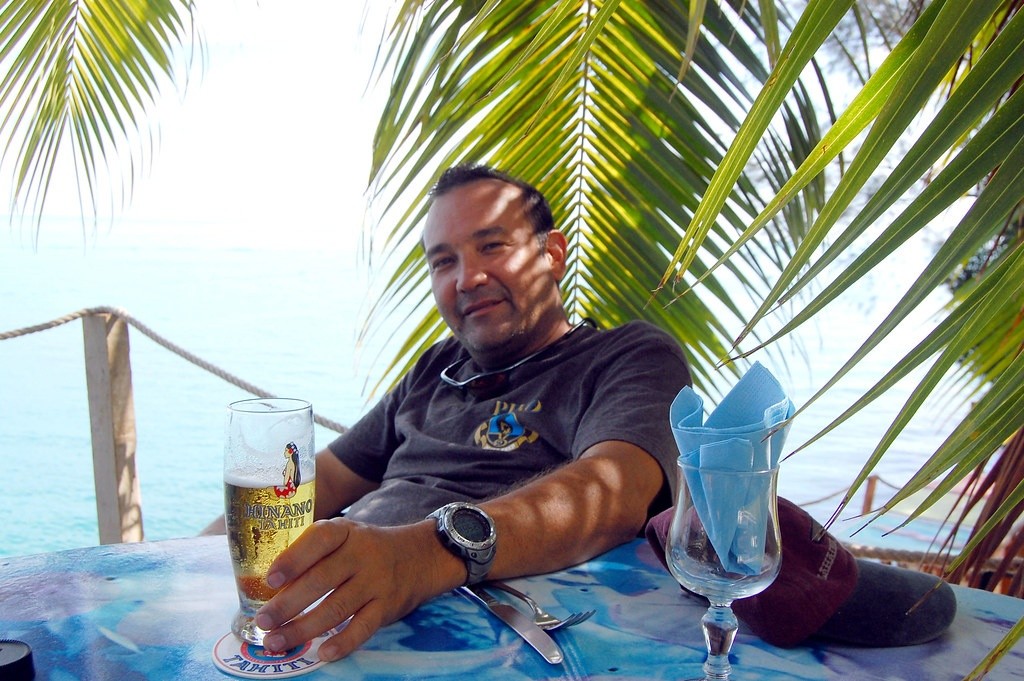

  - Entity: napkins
[668,360,796,578]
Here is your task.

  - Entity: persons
[197,165,691,662]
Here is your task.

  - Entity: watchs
[423,502,498,588]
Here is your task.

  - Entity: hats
[644,492,956,650]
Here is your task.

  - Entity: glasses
[439,317,596,402]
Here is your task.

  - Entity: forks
[488,581,596,633]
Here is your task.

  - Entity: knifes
[455,586,564,664]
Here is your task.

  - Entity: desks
[1,535,1024,681]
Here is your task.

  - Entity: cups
[222,398,316,646]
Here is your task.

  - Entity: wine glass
[665,458,782,680]
